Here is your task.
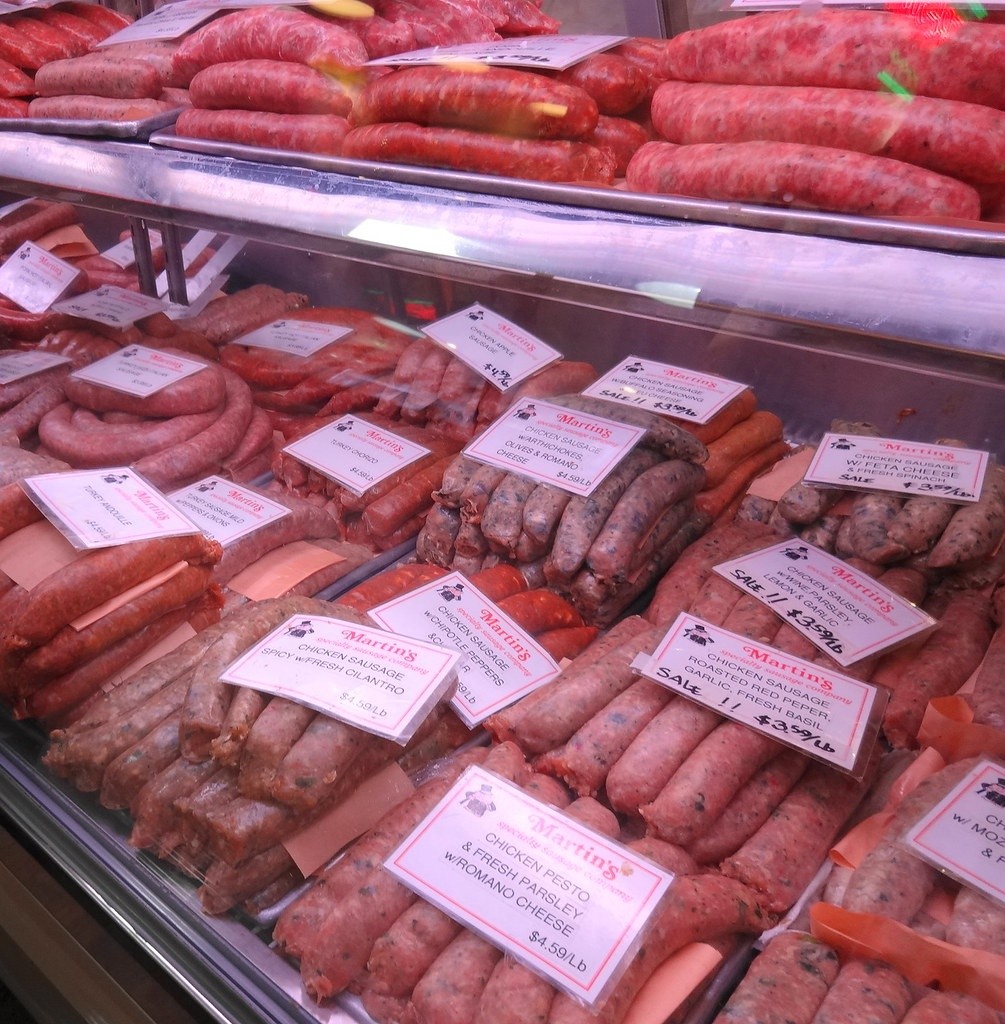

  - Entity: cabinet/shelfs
[0,132,1005,1024]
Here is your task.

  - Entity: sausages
[0,0,1005,235]
[1,194,1005,1024]
